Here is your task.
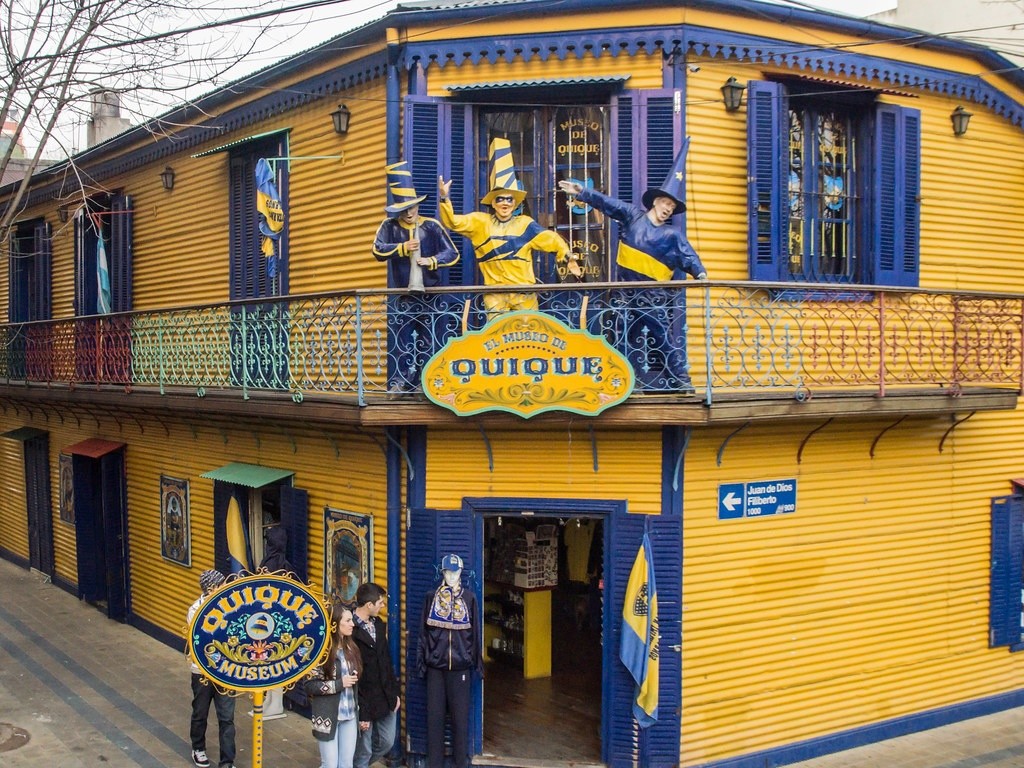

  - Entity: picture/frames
[321,504,375,600]
[58,453,75,524]
[160,474,192,568]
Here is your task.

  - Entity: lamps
[721,76,747,111]
[950,105,974,134]
[159,165,175,190]
[56,207,68,223]
[329,101,352,133]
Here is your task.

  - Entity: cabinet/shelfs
[484,579,558,681]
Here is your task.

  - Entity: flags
[96,226,112,314]
[254,158,287,279]
[223,488,254,585]
[618,534,659,730]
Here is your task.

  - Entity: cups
[491,637,514,653]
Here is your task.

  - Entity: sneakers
[222,763,236,768]
[192,750,210,767]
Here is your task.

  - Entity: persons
[353,582,400,768]
[183,570,237,768]
[301,603,368,768]
[557,136,709,395]
[246,525,292,721]
[370,161,461,401]
[417,553,487,768]
[438,137,584,327]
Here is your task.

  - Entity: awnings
[0,425,51,442]
[59,437,129,459]
[199,462,294,488]
[189,127,292,158]
[57,185,127,208]
[761,71,921,99]
[2,215,44,228]
[442,74,632,92]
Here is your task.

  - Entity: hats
[440,554,464,573]
[199,570,225,596]
